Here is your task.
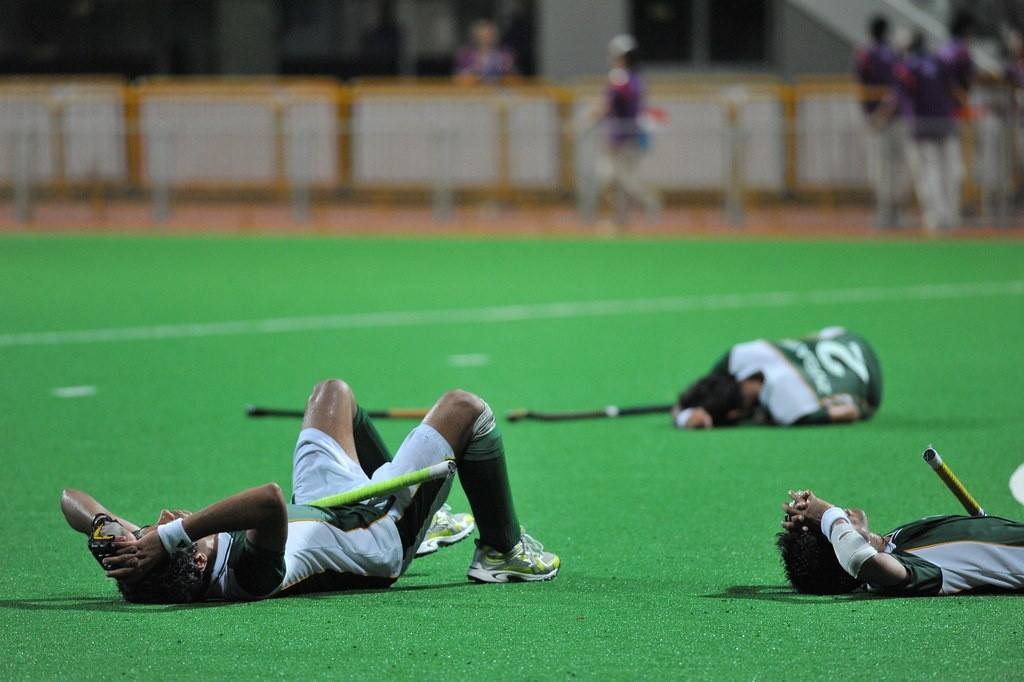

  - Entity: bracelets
[821,507,851,541]
[157,518,193,556]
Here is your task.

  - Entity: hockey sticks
[308,458,455,508]
[241,403,441,420]
[921,445,988,515]
[505,403,676,423]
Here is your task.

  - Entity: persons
[775,463,1024,597]
[60,376,561,606]
[563,33,667,223]
[854,4,1024,235]
[671,326,883,429]
[347,0,517,86]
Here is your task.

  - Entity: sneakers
[466,525,560,583]
[416,503,474,555]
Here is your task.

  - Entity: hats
[606,32,634,60]
